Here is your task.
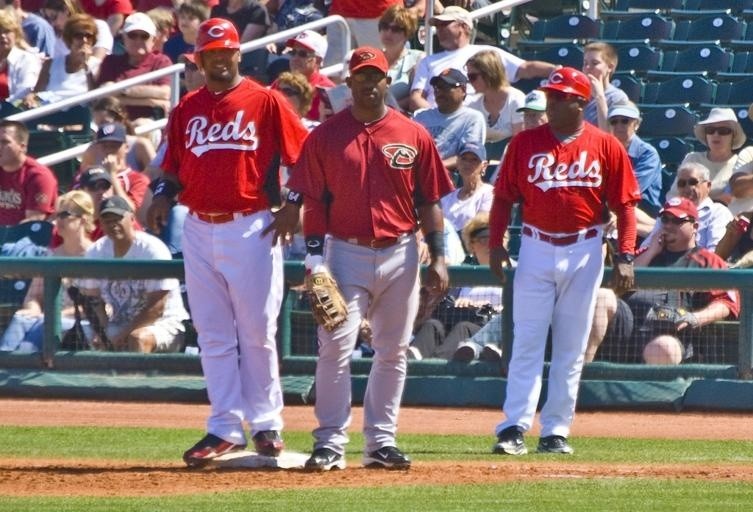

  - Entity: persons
[287,43,458,474]
[583,43,663,234]
[488,65,643,454]
[142,17,311,466]
[1,1,554,371]
[583,108,752,364]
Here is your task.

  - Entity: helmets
[536,67,591,102]
[193,18,240,53]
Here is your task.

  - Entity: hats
[429,6,473,31]
[285,30,328,59]
[472,228,490,238]
[78,168,111,184]
[693,107,747,150]
[121,12,156,37]
[95,123,125,143]
[98,196,131,215]
[607,99,640,119]
[457,141,486,162]
[515,89,547,113]
[429,68,467,91]
[658,196,698,222]
[348,47,388,76]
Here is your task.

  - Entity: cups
[733,214,750,237]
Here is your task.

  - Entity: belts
[522,226,597,245]
[189,208,256,224]
[357,237,396,251]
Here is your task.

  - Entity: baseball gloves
[303,272,349,333]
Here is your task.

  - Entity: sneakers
[453,346,477,360]
[304,448,346,470]
[251,431,282,456]
[494,431,527,455]
[406,346,422,360]
[478,345,501,360]
[360,446,410,469]
[182,433,246,465]
[535,435,573,454]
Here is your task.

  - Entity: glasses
[287,49,306,57]
[468,73,478,81]
[276,88,299,97]
[609,119,628,125]
[677,178,699,187]
[72,32,93,40]
[353,72,384,82]
[56,211,71,219]
[704,127,732,135]
[378,23,405,33]
[87,181,110,191]
[432,83,452,92]
[660,215,691,225]
[126,33,149,40]
[546,91,570,101]
